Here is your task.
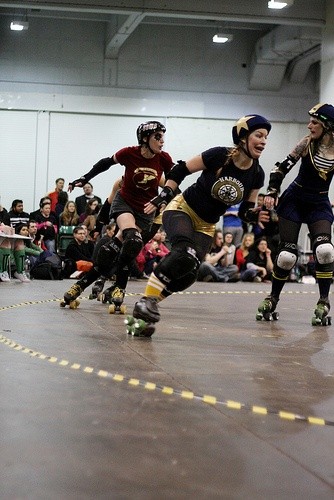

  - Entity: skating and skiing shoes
[89,276,104,299]
[99,284,116,304]
[110,287,126,314]
[61,280,86,309]
[312,297,332,326]
[256,295,280,320]
[124,296,160,336]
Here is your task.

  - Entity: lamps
[267,0,294,9]
[10,7,29,31]
[213,21,233,44]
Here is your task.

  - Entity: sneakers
[14,270,30,283]
[0,271,11,281]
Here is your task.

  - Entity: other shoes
[228,273,240,282]
[253,277,262,282]
[203,275,213,281]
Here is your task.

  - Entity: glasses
[76,233,85,235]
[92,204,97,206]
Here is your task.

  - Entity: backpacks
[31,260,65,280]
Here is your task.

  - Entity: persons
[258,103,334,317]
[132,114,272,322]
[64,121,182,304]
[132,194,279,283]
[0,177,118,283]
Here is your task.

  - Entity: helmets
[136,121,166,143]
[309,103,334,121]
[232,114,272,144]
